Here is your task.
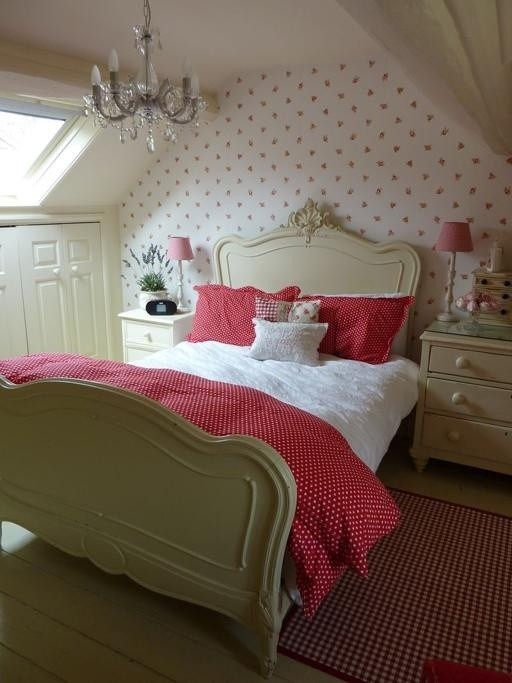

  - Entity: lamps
[433,221,474,322]
[166,236,194,308]
[82,0,208,153]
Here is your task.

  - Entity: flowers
[119,241,174,291]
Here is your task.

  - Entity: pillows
[245,317,328,367]
[293,295,416,365]
[254,295,321,324]
[185,283,301,347]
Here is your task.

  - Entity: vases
[139,290,169,312]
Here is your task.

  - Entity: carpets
[278,484,512,683]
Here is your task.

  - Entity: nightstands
[408,317,512,479]
[117,304,193,364]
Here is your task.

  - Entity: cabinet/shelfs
[472,269,512,327]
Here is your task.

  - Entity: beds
[0,197,422,679]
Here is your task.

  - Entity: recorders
[146,299,177,315]
